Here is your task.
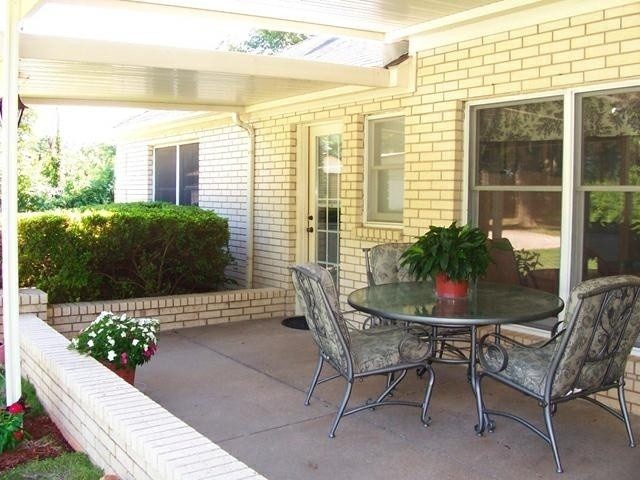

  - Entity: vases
[97,357,137,384]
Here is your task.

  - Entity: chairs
[364,241,474,384]
[470,271,640,479]
[289,258,438,438]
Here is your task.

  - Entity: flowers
[64,309,162,377]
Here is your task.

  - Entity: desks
[347,281,565,437]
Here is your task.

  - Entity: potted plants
[396,217,499,298]
